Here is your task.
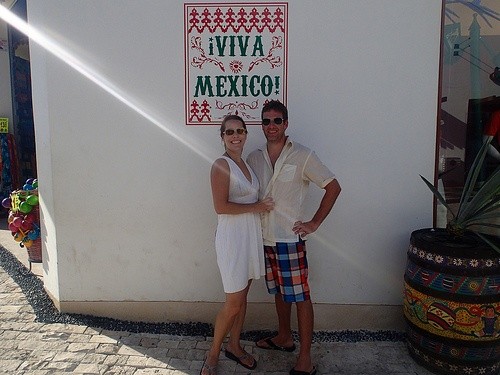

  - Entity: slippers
[256,338,296,352]
[225,348,257,370]
[199,356,216,375]
[288,365,317,375]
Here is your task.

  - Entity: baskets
[24,237,42,263]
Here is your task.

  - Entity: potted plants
[403,136,500,375]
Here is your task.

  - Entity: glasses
[262,117,284,126]
[223,128,246,136]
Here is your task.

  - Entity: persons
[198,115,276,375]
[246,100,343,375]
